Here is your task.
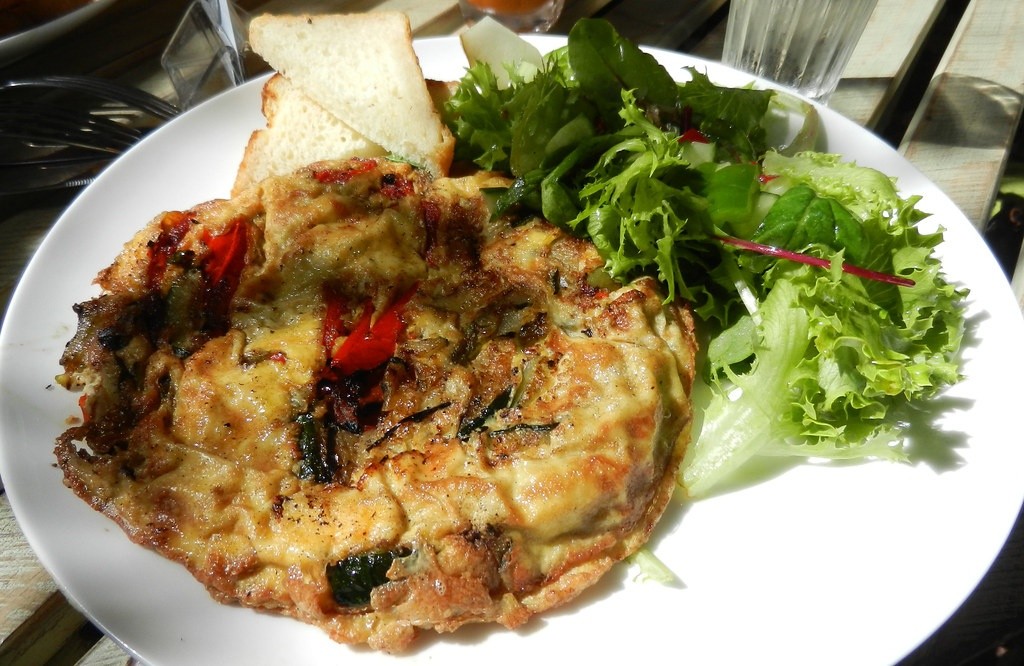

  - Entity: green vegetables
[442,13,979,499]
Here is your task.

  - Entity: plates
[0,0,120,68]
[0,30,1024,666]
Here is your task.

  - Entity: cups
[721,0,879,108]
[459,0,564,37]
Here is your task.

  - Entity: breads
[229,11,455,197]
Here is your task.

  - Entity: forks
[0,70,182,121]
[0,98,141,156]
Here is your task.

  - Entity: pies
[57,156,700,653]
